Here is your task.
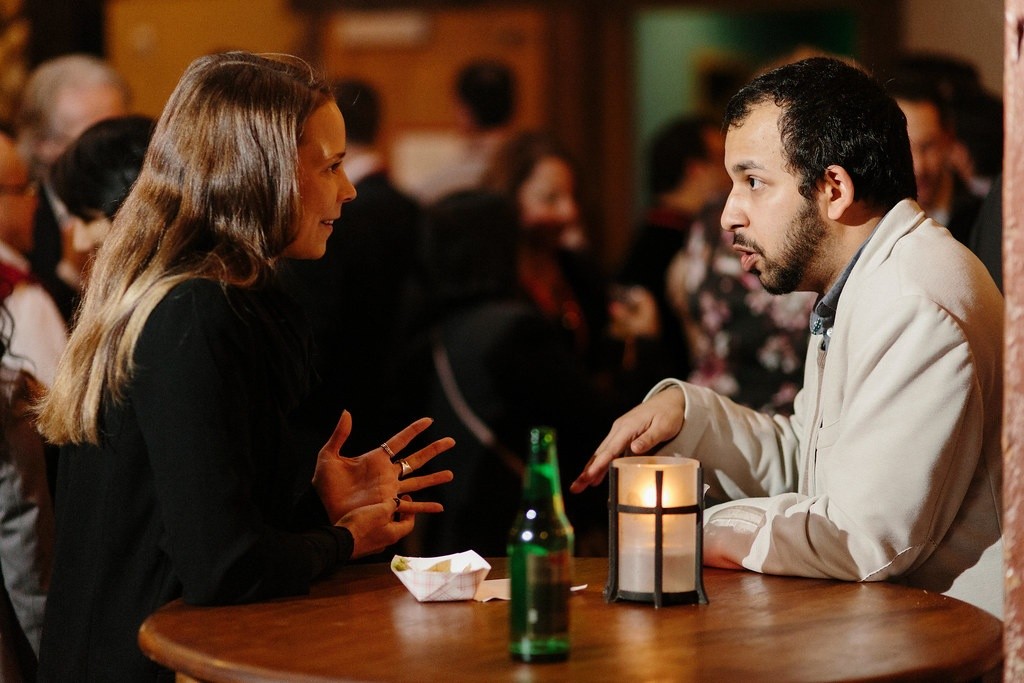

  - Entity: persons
[2,52,1004,657]
[571,56,1009,621]
[31,53,456,683]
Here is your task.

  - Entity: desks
[139,558,1005,683]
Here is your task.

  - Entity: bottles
[509,426,574,663]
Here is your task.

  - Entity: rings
[395,458,413,477]
[380,442,397,459]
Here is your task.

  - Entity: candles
[603,455,710,609]
